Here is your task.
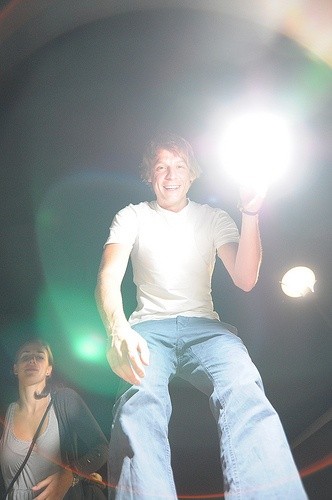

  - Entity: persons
[0,338,110,499]
[93,134,308,500]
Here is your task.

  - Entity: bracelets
[240,207,258,216]
